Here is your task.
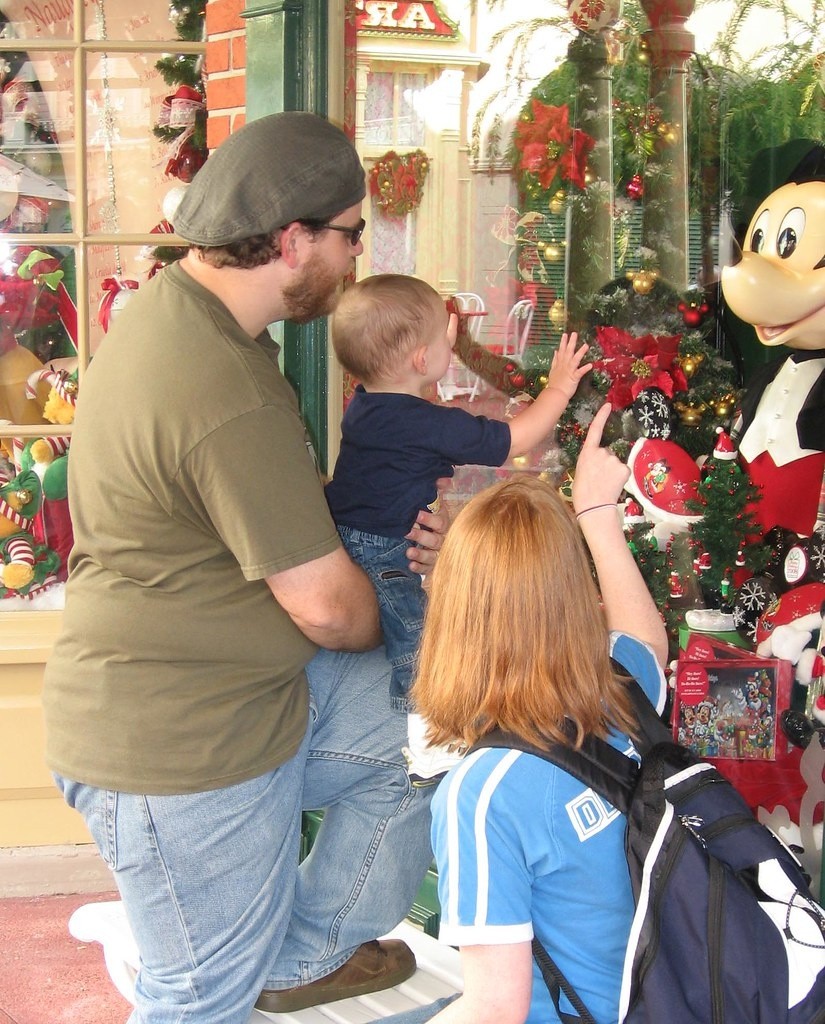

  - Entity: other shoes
[253,939,417,1012]
[402,737,469,789]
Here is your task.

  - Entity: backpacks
[463,652,825,1024]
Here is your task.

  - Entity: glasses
[327,218,366,246]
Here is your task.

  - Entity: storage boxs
[671,633,794,761]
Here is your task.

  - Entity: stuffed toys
[0,369,79,593]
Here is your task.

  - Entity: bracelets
[576,504,617,521]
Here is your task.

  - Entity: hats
[172,110,366,245]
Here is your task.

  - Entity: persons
[409,401,670,1024]
[324,272,592,787]
[44,114,450,1024]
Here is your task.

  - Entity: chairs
[435,292,538,402]
